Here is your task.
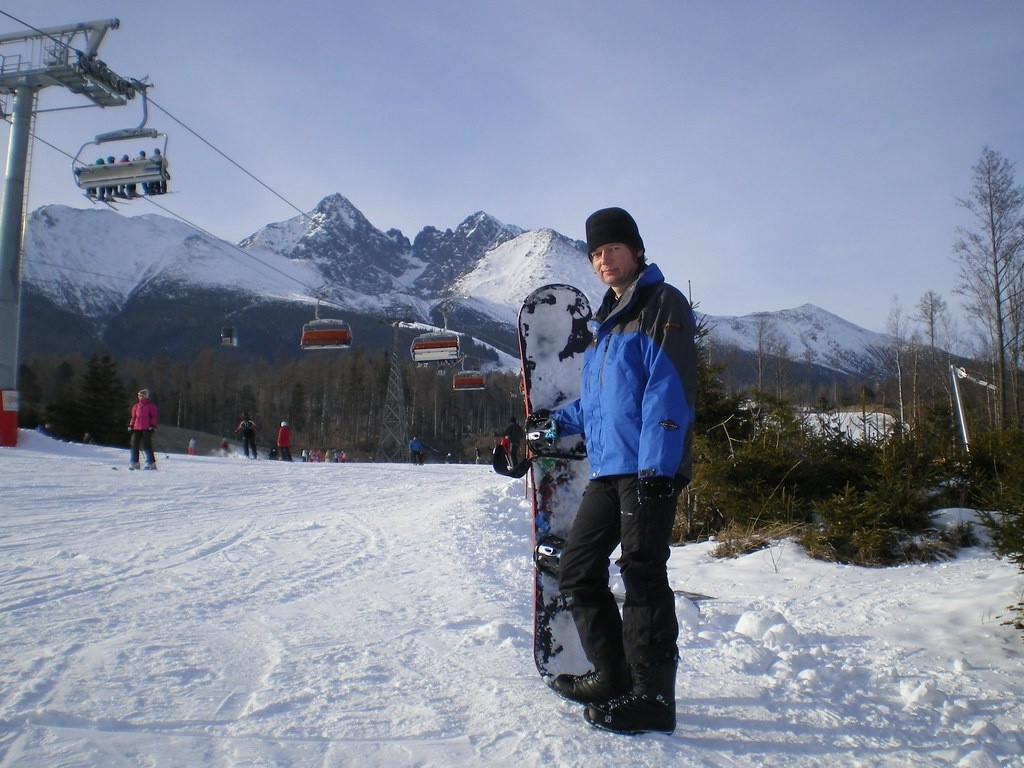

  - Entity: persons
[87,163,97,195]
[149,148,162,193]
[325,448,345,463]
[83,433,94,443]
[235,412,260,458]
[445,452,453,464]
[106,156,127,197]
[136,150,148,194]
[268,447,278,460]
[277,421,292,461]
[95,158,112,200]
[409,434,423,466]
[127,389,158,467]
[189,438,198,455]
[302,448,324,462]
[220,438,229,448]
[118,154,135,201]
[524,206,696,736]
[493,416,525,467]
[475,446,480,463]
[40,423,53,434]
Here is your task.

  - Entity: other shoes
[144,462,157,470]
[129,463,140,470]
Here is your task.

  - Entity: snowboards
[516,283,596,687]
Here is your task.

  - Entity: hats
[139,389,149,399]
[281,422,286,426]
[586,208,645,264]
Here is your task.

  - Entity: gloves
[128,426,133,432]
[638,477,677,505]
[149,426,157,432]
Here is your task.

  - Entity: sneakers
[553,670,629,705]
[583,692,677,736]
